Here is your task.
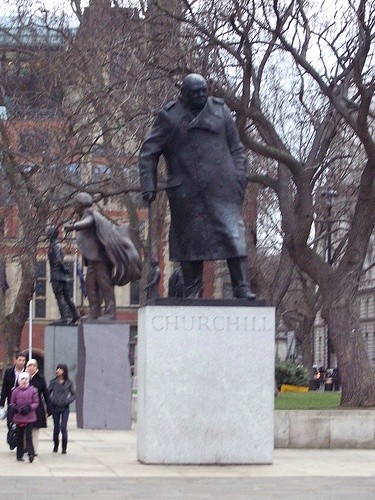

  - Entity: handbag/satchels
[7,423,17,450]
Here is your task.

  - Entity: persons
[0,353,27,450]
[16,359,55,456]
[9,372,39,463]
[168,266,184,300]
[138,73,258,300]
[313,367,342,392]
[143,257,161,300]
[45,228,80,325]
[47,363,76,454]
[56,193,142,322]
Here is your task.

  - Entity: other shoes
[17,455,24,461]
[29,454,34,462]
[62,445,66,454]
[53,445,59,452]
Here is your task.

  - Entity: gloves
[20,405,31,415]
[47,407,54,416]
[11,406,19,414]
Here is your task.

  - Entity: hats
[26,359,37,367]
[18,372,30,380]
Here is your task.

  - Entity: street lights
[318,185,339,369]
